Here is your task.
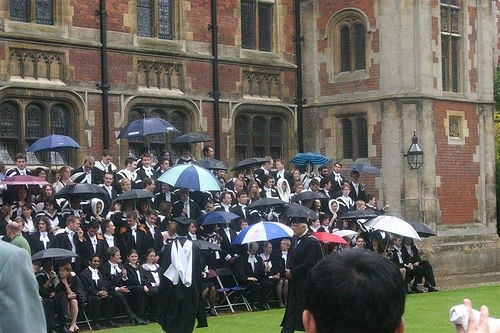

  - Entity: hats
[173,215,195,237]
[289,217,309,224]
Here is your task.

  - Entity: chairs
[205,268,253,315]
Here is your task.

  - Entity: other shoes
[132,317,148,325]
[91,322,101,330]
[61,326,73,333]
[278,303,286,308]
[407,287,412,294]
[411,286,423,293]
[50,329,57,333]
[59,313,72,321]
[250,303,258,311]
[261,303,267,310]
[424,282,432,289]
[69,325,80,332]
[52,322,60,327]
[105,320,122,328]
[210,307,218,316]
[429,288,439,292]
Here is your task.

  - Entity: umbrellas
[0,112,436,273]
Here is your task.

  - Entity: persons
[0,145,500,333]
[157,215,202,333]
[280,218,323,332]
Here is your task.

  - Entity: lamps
[404,130,423,169]
